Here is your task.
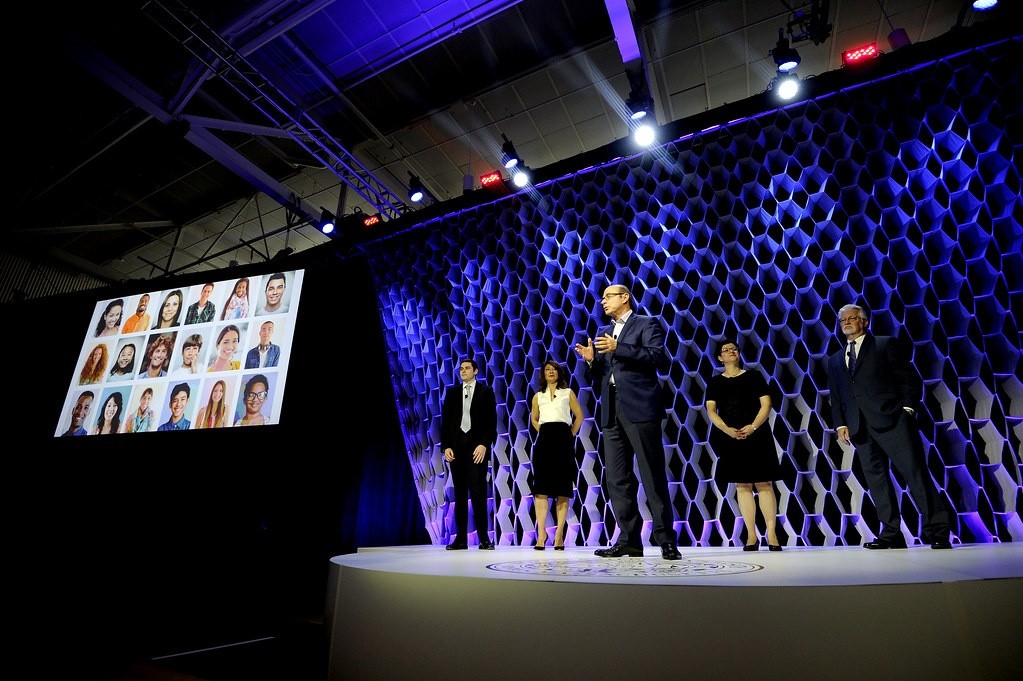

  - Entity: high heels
[534,533,548,550]
[554,543,565,551]
[743,537,760,551]
[768,544,783,551]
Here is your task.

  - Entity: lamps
[318,206,336,236]
[407,170,424,202]
[876,0,912,51]
[769,27,803,70]
[500,133,518,169]
[463,103,474,195]
[625,89,648,121]
[512,160,532,188]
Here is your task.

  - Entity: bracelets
[752,425,756,430]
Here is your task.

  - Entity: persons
[575,285,682,560]
[531,361,584,550]
[152,283,216,330]
[61,390,123,437]
[76,343,136,385]
[206,321,281,372]
[194,374,271,429]
[440,360,496,550]
[219,272,289,321]
[705,338,784,551]
[827,303,953,549]
[93,294,151,338]
[124,383,191,433]
[138,333,203,379]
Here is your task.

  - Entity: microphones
[847,352,851,356]
[554,395,556,398]
[614,335,617,339]
[465,395,468,398]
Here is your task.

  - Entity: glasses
[720,346,738,353]
[600,293,625,301]
[245,392,267,401]
[839,315,861,323]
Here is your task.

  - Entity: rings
[737,438,739,439]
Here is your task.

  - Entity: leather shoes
[662,544,682,559]
[479,539,495,549]
[594,543,643,557]
[446,541,467,550]
[864,539,907,549]
[931,540,950,548]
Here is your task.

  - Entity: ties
[461,385,472,433]
[849,342,857,378]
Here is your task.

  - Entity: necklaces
[259,344,270,351]
[725,369,741,376]
